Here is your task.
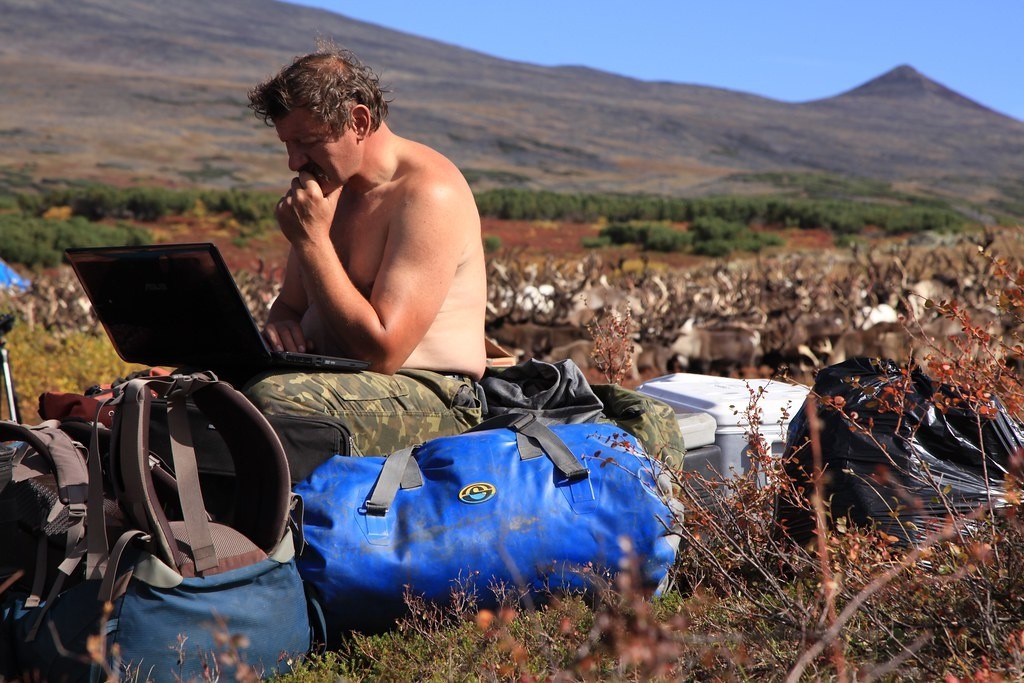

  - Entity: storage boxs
[659,445,724,599]
[633,371,812,522]
[662,406,717,450]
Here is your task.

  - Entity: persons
[241,51,487,461]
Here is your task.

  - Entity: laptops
[65,242,371,371]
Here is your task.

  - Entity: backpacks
[46,377,313,683]
[0,420,91,670]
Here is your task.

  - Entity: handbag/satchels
[289,412,685,639]
